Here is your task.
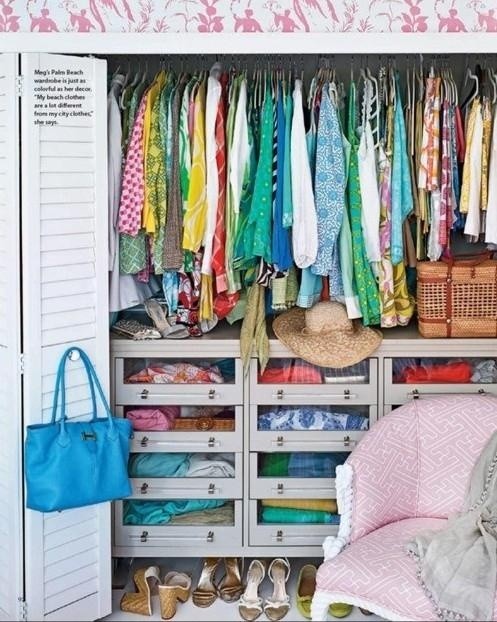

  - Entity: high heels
[112,298,218,339]
[119,558,372,621]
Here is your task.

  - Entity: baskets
[417,260,497,337]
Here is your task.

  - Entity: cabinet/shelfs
[109,319,497,560]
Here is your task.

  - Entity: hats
[273,301,382,368]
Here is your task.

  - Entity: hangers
[111,54,497,115]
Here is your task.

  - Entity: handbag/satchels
[25,347,132,512]
